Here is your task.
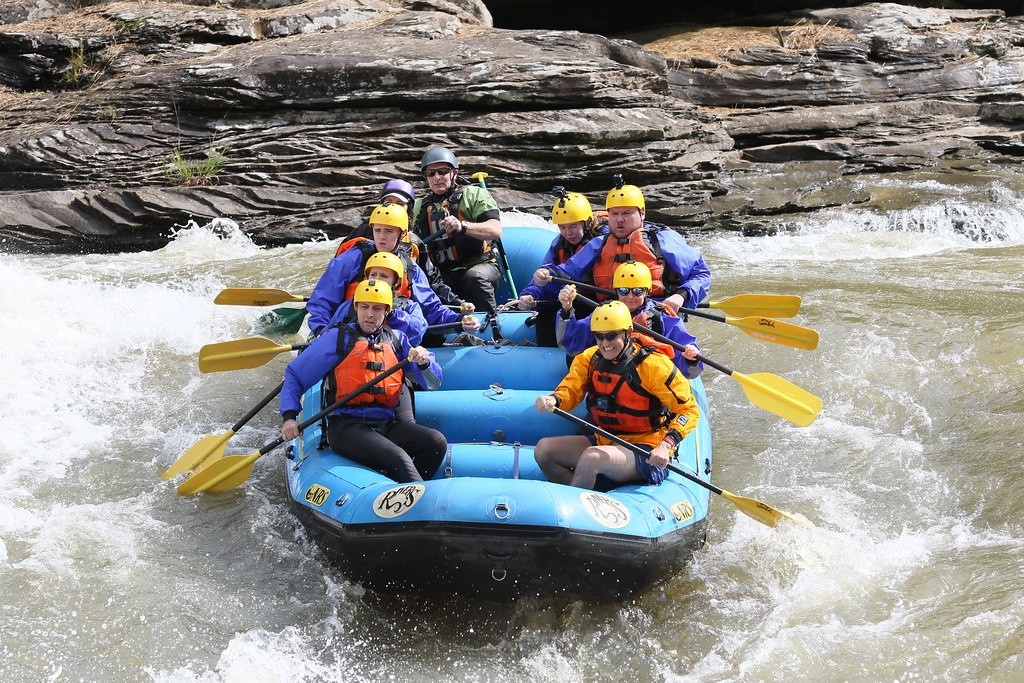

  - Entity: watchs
[461,221,467,233]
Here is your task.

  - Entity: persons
[534,300,699,490]
[409,147,503,313]
[519,193,608,346]
[554,259,703,379]
[533,185,711,314]
[278,178,479,484]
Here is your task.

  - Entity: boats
[282,227,713,575]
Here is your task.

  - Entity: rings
[423,357,425,360]
[449,224,453,227]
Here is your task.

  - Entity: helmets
[363,253,404,289]
[354,279,393,314]
[552,193,594,224]
[606,185,645,212]
[368,202,408,242]
[590,300,633,332]
[381,179,415,210]
[613,261,653,293]
[421,147,459,172]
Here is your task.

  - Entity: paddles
[551,406,798,529]
[162,379,285,480]
[176,348,416,495]
[569,283,823,428]
[528,293,802,319]
[422,226,447,243]
[543,270,819,352]
[213,286,470,318]
[198,316,473,375]
[473,171,518,298]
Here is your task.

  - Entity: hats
[382,191,411,203]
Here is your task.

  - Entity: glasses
[424,166,454,177]
[616,288,646,297]
[593,330,625,341]
